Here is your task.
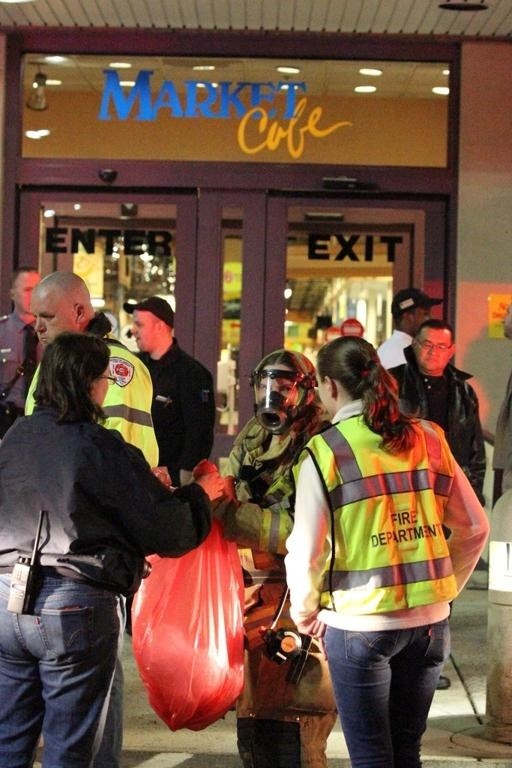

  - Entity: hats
[389,287,445,326]
[122,296,177,331]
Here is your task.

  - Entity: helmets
[249,348,319,435]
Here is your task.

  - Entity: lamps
[24,56,54,111]
